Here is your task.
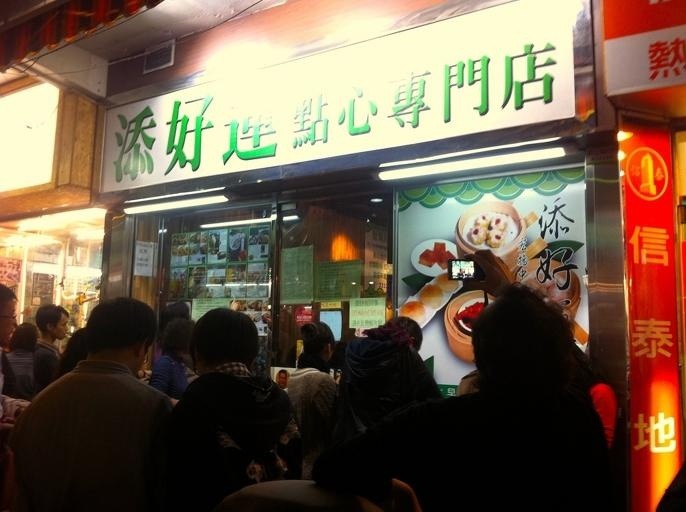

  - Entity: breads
[398,299,427,326]
[435,272,460,294]
[419,284,443,310]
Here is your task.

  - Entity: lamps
[371,136,578,181]
[116,186,233,215]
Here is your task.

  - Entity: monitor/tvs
[318,309,342,342]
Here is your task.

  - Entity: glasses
[1,311,21,320]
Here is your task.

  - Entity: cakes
[471,213,506,249]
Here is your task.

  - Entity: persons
[1,249,630,511]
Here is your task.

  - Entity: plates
[410,239,462,278]
[463,212,519,253]
[395,278,465,330]
[523,269,570,305]
[457,297,495,333]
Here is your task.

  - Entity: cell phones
[448,257,484,282]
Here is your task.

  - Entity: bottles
[329,368,335,379]
[336,367,341,385]
